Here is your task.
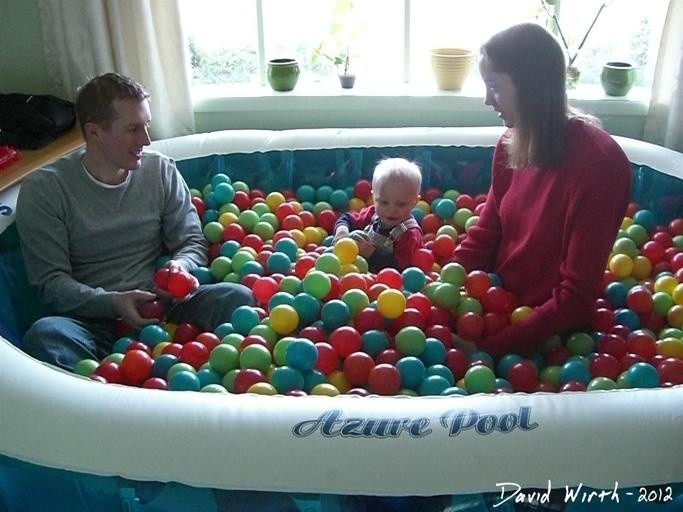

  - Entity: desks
[0,122,86,192]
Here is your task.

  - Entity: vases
[430,48,474,91]
[266,58,300,91]
[601,61,637,95]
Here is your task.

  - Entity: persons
[16,71,261,374]
[446,22,632,364]
[330,158,428,275]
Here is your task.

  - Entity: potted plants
[311,0,361,89]
[535,0,608,91]
